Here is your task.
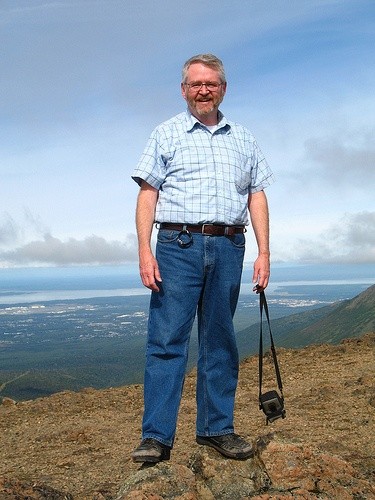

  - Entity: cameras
[259,389,285,422]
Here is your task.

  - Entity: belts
[161,221,246,236]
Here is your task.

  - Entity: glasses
[183,79,225,94]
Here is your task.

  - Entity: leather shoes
[130,437,171,463]
[197,430,254,459]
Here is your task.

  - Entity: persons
[130,53,276,463]
